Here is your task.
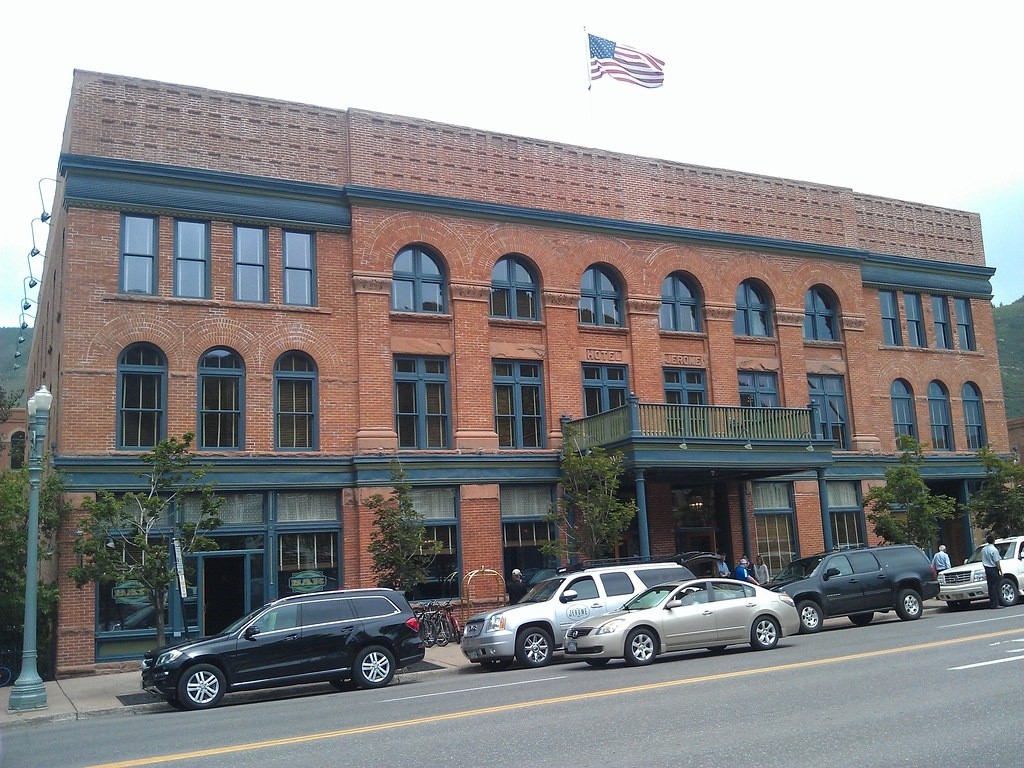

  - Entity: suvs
[460,562,700,671]
[937,535,1024,611]
[758,543,941,634]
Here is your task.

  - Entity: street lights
[8,384,53,713]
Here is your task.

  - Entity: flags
[589,33,665,88]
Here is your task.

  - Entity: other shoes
[990,606,1005,609]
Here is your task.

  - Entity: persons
[756,556,769,584]
[981,536,1005,609]
[507,569,527,605]
[733,559,760,585]
[684,589,699,605]
[932,545,951,600]
[718,553,731,577]
[742,555,760,583]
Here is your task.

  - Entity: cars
[141,588,426,711]
[564,577,804,667]
[507,568,558,592]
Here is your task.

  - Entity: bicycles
[410,598,461,649]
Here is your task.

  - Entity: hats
[512,569,522,575]
[740,559,747,563]
